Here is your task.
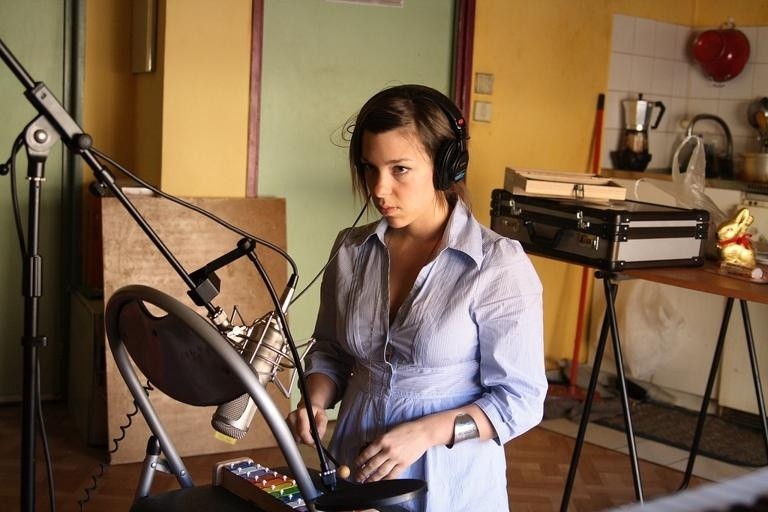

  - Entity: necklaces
[387,231,442,322]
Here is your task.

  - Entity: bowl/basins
[690,22,751,87]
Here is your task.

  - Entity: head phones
[400,84,469,190]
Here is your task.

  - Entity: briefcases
[490,189,710,271]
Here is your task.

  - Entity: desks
[557,263,767,511]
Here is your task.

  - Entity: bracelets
[447,415,479,448]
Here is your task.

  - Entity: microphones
[211,275,299,440]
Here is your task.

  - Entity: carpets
[587,396,766,466]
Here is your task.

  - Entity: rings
[359,464,373,478]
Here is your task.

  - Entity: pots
[738,152,768,187]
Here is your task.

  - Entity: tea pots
[621,93,665,132]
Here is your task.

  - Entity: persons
[284,83,549,512]
